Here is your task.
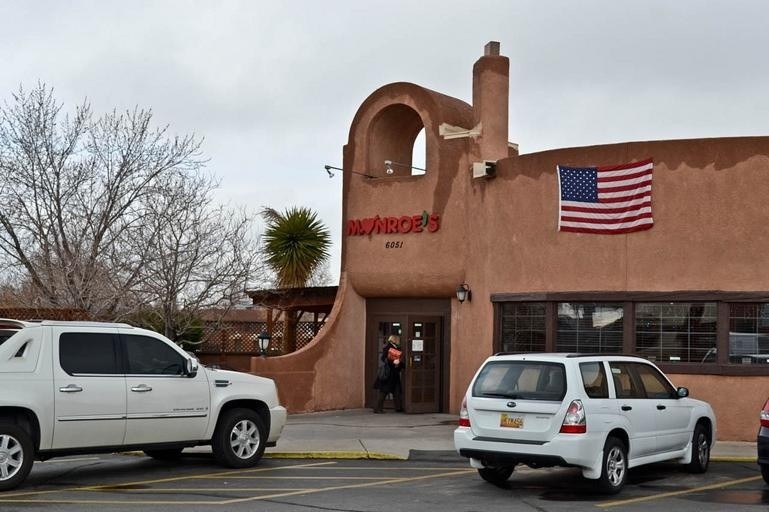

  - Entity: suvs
[451,353,719,492]
[727,331,769,364]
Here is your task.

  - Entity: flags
[556,158,656,235]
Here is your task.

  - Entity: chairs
[544,369,624,397]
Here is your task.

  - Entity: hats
[390,327,402,336]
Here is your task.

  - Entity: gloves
[393,359,401,365]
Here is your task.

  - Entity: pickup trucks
[0,320,289,492]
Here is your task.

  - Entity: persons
[372,334,406,414]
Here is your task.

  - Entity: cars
[757,396,768,485]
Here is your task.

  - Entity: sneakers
[395,407,406,413]
[373,408,386,414]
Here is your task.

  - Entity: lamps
[257,331,270,356]
[385,160,426,175]
[325,165,379,178]
[456,283,472,304]
